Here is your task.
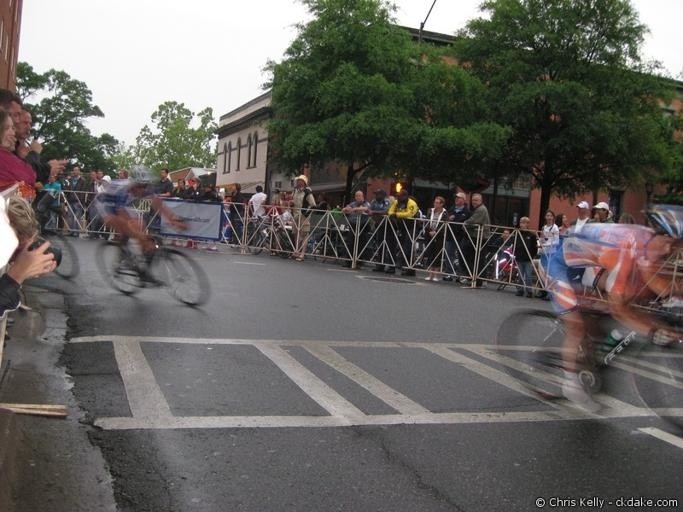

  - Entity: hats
[577,201,588,209]
[456,192,466,200]
[373,189,386,195]
[295,175,308,185]
[593,202,609,211]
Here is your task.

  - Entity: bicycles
[246,214,293,259]
[31,188,81,280]
[496,285,682,434]
[92,215,211,308]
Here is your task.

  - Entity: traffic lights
[394,181,407,193]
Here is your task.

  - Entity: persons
[0,89,683,413]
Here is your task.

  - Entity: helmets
[643,205,683,240]
[131,166,151,184]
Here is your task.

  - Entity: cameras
[27,240,62,272]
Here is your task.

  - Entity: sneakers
[425,275,482,286]
[516,290,549,301]
[373,268,416,276]
[342,262,350,266]
